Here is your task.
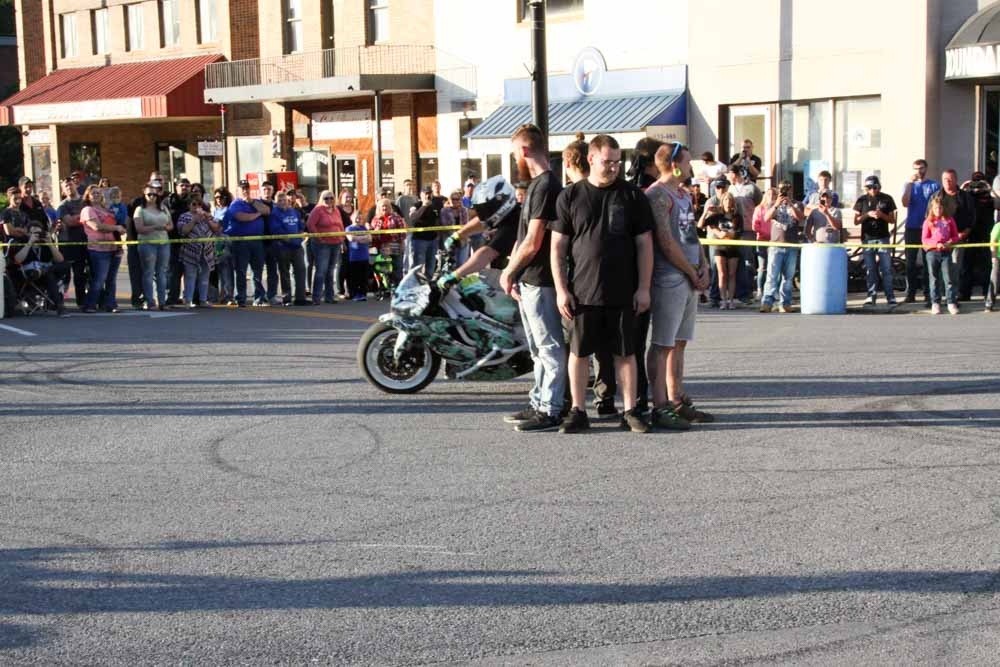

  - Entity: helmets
[470,176,516,228]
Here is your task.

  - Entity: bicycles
[844,248,907,291]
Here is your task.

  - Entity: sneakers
[599,403,618,418]
[652,405,691,429]
[674,391,713,422]
[504,404,558,432]
[558,407,589,434]
[622,407,649,432]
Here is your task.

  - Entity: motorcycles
[357,248,595,397]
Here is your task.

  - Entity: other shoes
[698,291,993,316]
[54,291,365,318]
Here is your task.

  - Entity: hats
[714,179,731,187]
[19,176,33,185]
[865,175,880,187]
[238,180,248,187]
[821,189,832,198]
[691,177,700,185]
[421,187,432,193]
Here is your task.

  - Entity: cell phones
[35,232,46,238]
[743,151,747,159]
[821,200,825,208]
[711,207,722,211]
[197,207,203,213]
[782,191,787,196]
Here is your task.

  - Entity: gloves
[444,232,461,256]
[436,271,459,292]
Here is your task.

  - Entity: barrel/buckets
[799,244,847,315]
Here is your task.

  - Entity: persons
[853,176,898,307]
[0,122,1000,434]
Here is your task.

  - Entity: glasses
[215,196,221,198]
[669,141,681,165]
[193,203,202,206]
[593,157,623,168]
[144,192,158,197]
[324,197,333,200]
